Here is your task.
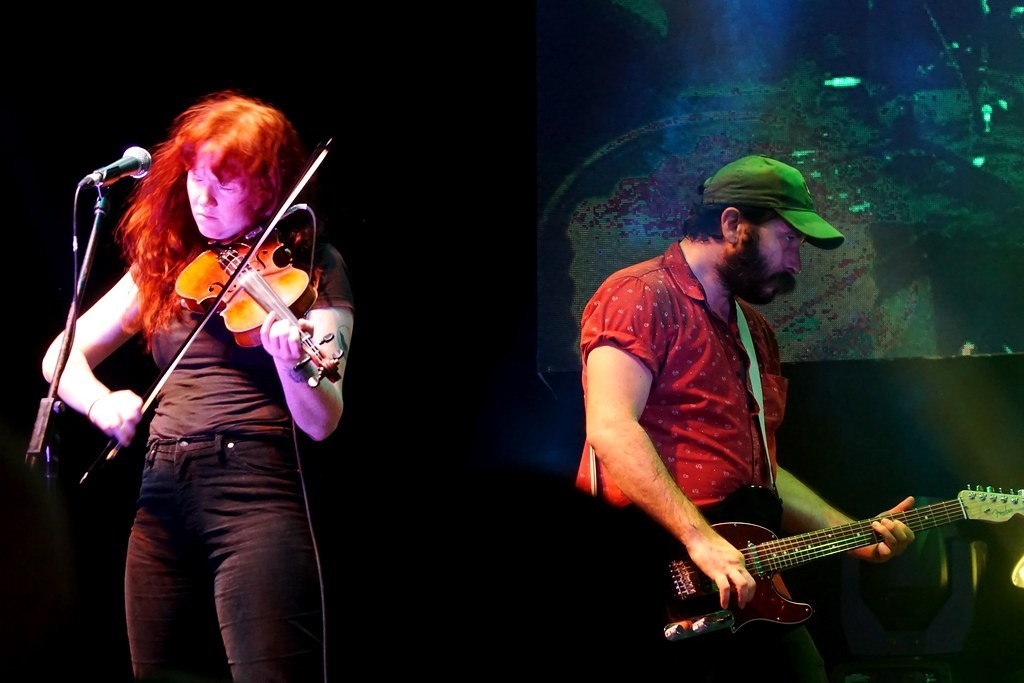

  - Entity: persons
[577,153,915,683]
[42,89,354,683]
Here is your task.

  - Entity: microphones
[82,146,153,191]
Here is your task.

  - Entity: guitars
[662,483,1024,637]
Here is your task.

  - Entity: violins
[174,213,348,390]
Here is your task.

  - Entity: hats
[702,155,846,251]
